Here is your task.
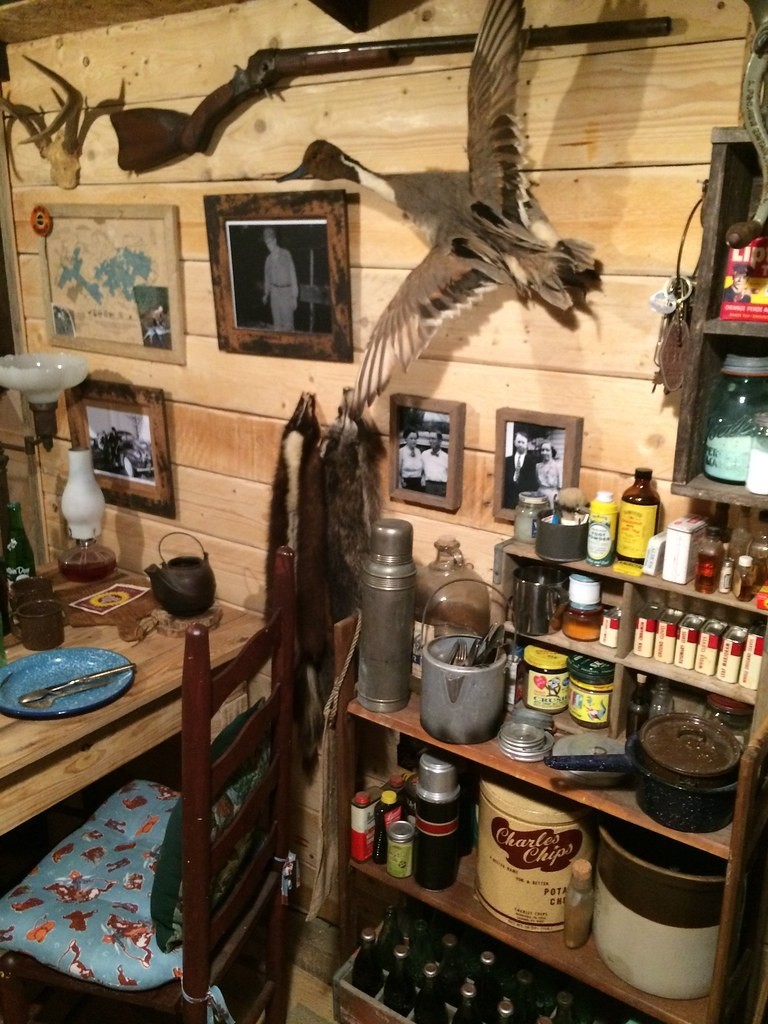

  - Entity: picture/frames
[387,392,467,511]
[202,188,354,363]
[63,378,177,521]
[492,407,583,521]
[37,202,188,367]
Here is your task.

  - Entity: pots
[545,712,739,832]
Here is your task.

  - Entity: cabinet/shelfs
[488,537,768,747]
[669,125,768,509]
[331,613,768,1024]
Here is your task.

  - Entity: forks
[24,681,112,708]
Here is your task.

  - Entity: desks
[0,560,266,840]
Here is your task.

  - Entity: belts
[271,283,291,288]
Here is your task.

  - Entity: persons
[503,430,562,511]
[261,227,298,331]
[398,427,449,498]
[723,263,753,302]
[152,305,164,325]
[96,427,120,464]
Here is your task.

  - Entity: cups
[418,634,508,744]
[512,566,567,636]
[8,599,64,651]
[11,577,53,629]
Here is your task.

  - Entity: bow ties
[431,452,439,457]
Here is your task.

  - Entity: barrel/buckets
[474,779,597,934]
[592,823,727,1001]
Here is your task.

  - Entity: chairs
[0,544,295,1024]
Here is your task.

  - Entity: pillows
[149,697,271,954]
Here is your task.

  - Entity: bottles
[352,905,604,1024]
[695,527,722,594]
[746,413,768,496]
[562,605,603,642]
[616,468,660,565]
[705,693,752,748]
[568,653,615,729]
[564,858,595,949]
[568,574,603,610]
[712,502,768,601]
[514,492,551,543]
[373,791,403,865]
[586,491,618,567]
[704,354,768,485]
[626,673,649,738]
[523,644,568,714]
[411,535,490,696]
[4,502,36,624]
[387,820,415,877]
[649,676,672,718]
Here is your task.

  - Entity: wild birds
[277,0,598,424]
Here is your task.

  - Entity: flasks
[358,519,417,714]
[417,752,460,889]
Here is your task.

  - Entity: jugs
[144,532,215,616]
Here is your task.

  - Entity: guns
[107,13,676,174]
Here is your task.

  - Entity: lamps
[57,445,117,583]
[0,350,90,456]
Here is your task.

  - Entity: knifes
[18,662,135,704]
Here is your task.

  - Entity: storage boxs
[661,512,710,585]
[641,531,666,576]
[718,237,768,323]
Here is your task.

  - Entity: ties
[516,456,521,484]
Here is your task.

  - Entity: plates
[0,647,133,716]
